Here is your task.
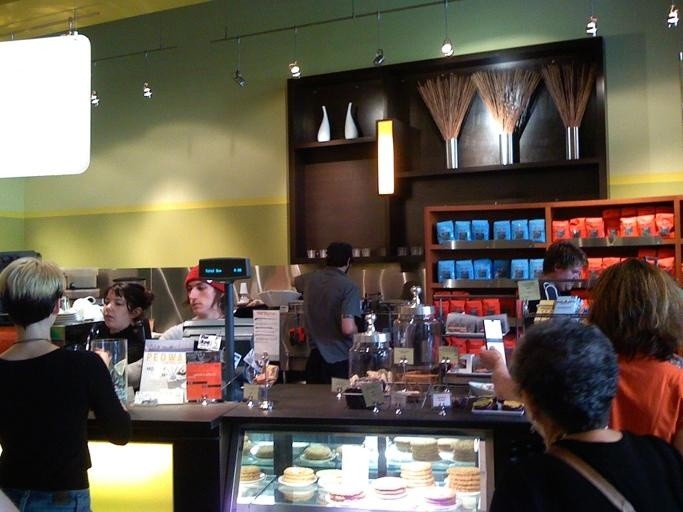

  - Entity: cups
[305,246,422,258]
[60,296,70,311]
[73,296,96,312]
[90,339,130,407]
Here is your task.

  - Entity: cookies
[240,436,273,483]
[278,438,480,507]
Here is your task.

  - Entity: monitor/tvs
[183,318,253,369]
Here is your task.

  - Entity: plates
[55,309,82,325]
[275,475,318,488]
[247,443,278,464]
[300,452,335,467]
[444,486,479,496]
[469,403,525,416]
[238,474,265,485]
[387,444,414,463]
[426,500,464,511]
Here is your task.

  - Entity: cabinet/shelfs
[285,35,607,262]
[424,195,682,362]
[220,382,496,512]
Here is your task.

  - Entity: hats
[184,264,226,293]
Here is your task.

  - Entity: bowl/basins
[257,289,302,308]
[468,381,494,396]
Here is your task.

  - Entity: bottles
[351,313,391,389]
[391,286,441,373]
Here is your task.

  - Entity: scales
[199,257,271,401]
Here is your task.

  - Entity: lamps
[0,0,94,184]
[374,118,400,198]
[90,0,681,108]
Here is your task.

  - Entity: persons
[294,242,369,384]
[488,319,683,512]
[0,256,132,512]
[72,281,154,393]
[128,264,238,390]
[528,239,588,325]
[478,257,683,456]
